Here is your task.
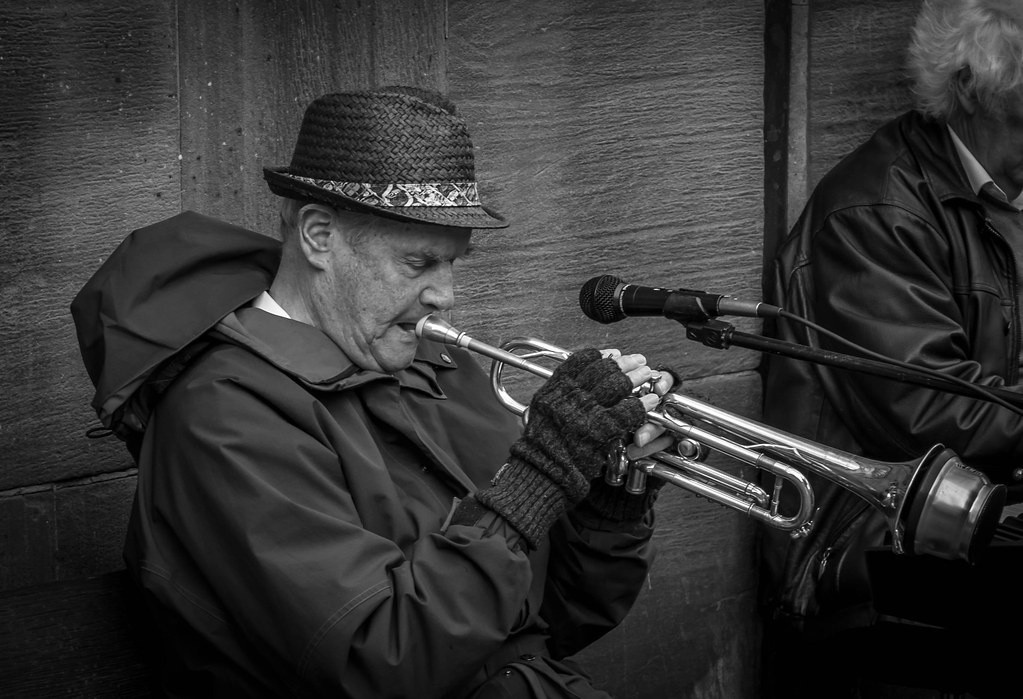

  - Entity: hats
[263,85,511,229]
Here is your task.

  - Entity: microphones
[579,274,784,324]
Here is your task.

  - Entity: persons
[748,1,1022,699]
[71,86,712,699]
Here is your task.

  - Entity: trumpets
[413,311,1007,565]
